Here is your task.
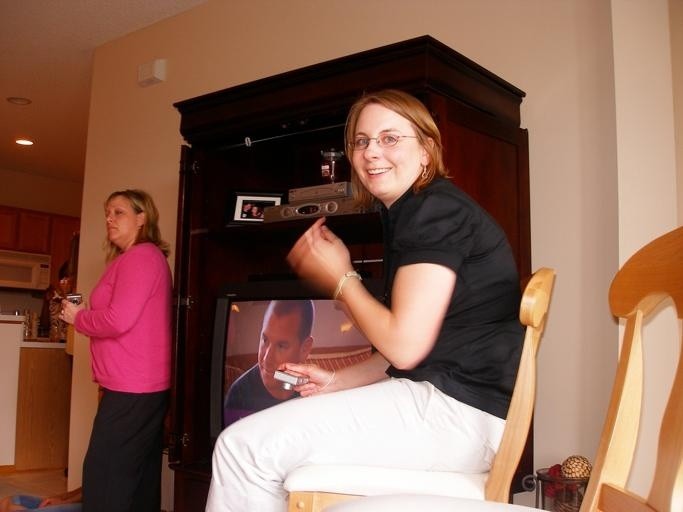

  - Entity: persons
[224,300,315,411]
[57,189,174,512]
[204,89,525,512]
[241,202,264,218]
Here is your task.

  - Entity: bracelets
[318,368,335,390]
[333,271,362,299]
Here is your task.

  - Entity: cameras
[66,293,82,305]
[273,369,310,391]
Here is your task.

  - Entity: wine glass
[320,147,344,182]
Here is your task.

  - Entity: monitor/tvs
[209,278,384,440]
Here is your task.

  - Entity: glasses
[347,133,417,149]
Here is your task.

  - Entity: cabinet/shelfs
[1,205,80,298]
[169,33,530,511]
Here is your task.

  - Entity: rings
[60,309,64,314]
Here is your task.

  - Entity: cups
[22,309,39,338]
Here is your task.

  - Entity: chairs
[321,227,683,512]
[283,268,554,512]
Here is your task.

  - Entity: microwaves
[0,249,52,290]
